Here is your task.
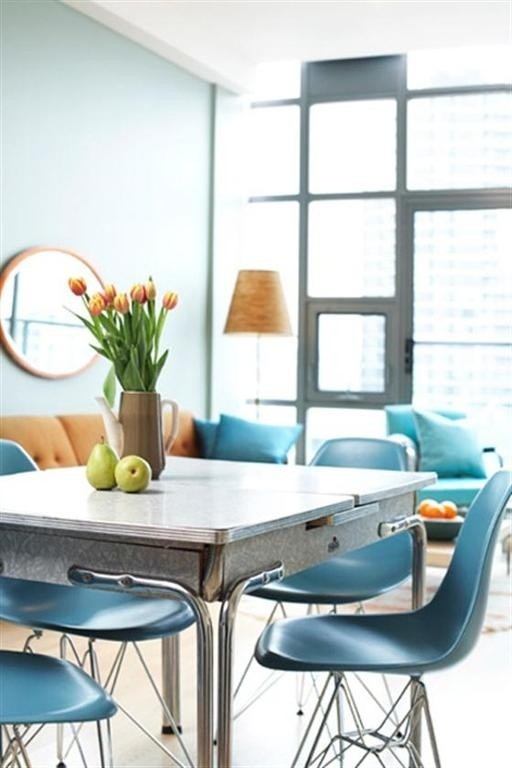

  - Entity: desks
[0,462,439,651]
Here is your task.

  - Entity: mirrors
[0,243,109,383]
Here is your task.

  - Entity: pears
[113,454,152,494]
[86,434,118,490]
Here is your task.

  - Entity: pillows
[415,411,493,480]
[217,414,303,462]
[191,418,215,460]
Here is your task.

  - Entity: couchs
[0,411,200,476]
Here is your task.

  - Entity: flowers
[68,272,180,390]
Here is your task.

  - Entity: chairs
[381,404,504,506]
[0,437,203,768]
[0,649,118,768]
[207,436,421,748]
[255,464,512,766]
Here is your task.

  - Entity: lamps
[222,270,294,414]
